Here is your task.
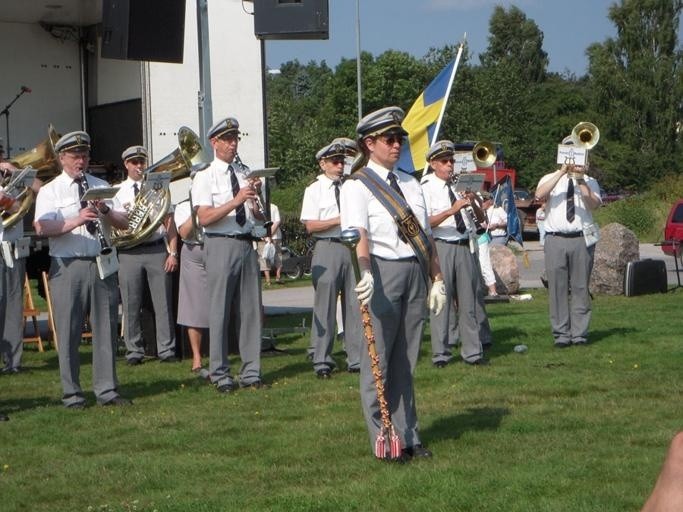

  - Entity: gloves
[429,280,447,317]
[353,272,374,306]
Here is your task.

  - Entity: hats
[425,140,454,163]
[206,117,240,139]
[355,106,407,139]
[561,135,572,145]
[331,137,356,153]
[315,142,346,160]
[54,130,91,152]
[121,145,147,162]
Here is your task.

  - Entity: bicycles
[279,227,316,280]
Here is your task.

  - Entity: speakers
[100,0,186,64]
[253,1,329,42]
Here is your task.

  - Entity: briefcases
[623,258,668,296]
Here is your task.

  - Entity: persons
[0,118,268,408]
[257,203,285,290]
[536,135,603,349]
[300,135,511,380]
[335,105,446,462]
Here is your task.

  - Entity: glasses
[331,160,344,165]
[377,137,405,146]
[440,159,455,164]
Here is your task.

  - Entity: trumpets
[566,121,600,179]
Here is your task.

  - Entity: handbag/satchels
[95,246,120,281]
[13,236,31,260]
[583,223,600,248]
[469,233,479,254]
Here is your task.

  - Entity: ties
[484,210,491,240]
[132,183,150,230]
[566,178,574,223]
[445,182,465,234]
[74,178,95,234]
[333,181,339,213]
[387,172,407,244]
[228,165,245,226]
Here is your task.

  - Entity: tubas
[108,126,207,250]
[0,122,59,229]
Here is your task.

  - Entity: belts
[545,231,582,238]
[206,233,251,239]
[139,239,163,247]
[434,237,468,246]
[399,257,418,262]
[491,236,505,237]
[315,237,340,242]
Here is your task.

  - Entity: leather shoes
[383,450,412,463]
[406,445,432,459]
[435,339,588,368]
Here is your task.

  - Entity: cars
[599,189,608,203]
[600,188,635,202]
[513,188,531,200]
[658,200,682,265]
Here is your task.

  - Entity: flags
[391,45,457,175]
